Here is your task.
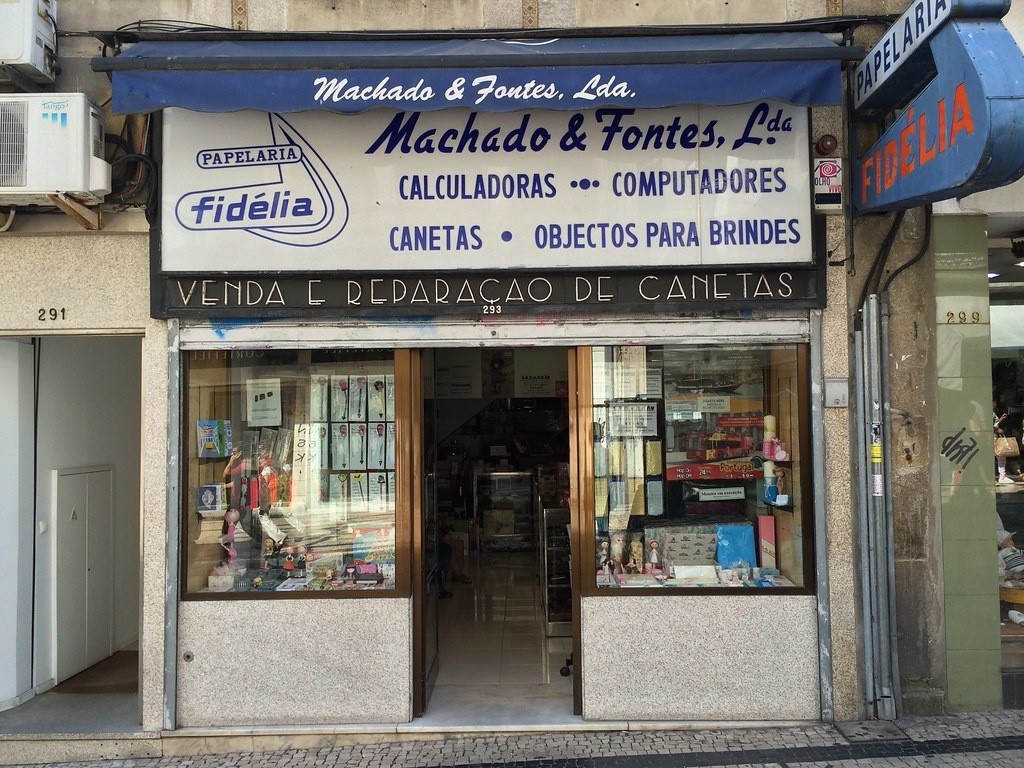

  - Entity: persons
[345,566,356,579]
[425,496,471,598]
[321,570,335,584]
[250,577,263,590]
[284,546,306,577]
[773,468,785,494]
[649,540,659,570]
[221,508,242,563]
[224,443,340,546]
[598,540,610,572]
[263,540,274,569]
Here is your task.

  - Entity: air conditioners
[0,0,58,86]
[0,91,112,212]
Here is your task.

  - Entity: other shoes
[998,477,1015,483]
[278,535,288,545]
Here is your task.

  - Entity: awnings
[90,31,847,115]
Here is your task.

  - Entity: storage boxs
[661,558,723,579]
[756,514,776,568]
[208,573,241,587]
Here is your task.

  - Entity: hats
[997,530,1017,546]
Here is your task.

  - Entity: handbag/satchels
[993,433,1020,457]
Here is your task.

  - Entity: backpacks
[259,474,271,515]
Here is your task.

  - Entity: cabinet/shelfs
[537,495,573,639]
[759,457,796,513]
[473,472,536,551]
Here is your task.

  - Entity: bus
[686,431,754,462]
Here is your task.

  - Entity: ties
[378,389,383,418]
[360,435,364,464]
[319,388,325,421]
[379,439,383,466]
[342,436,346,468]
[357,388,362,418]
[320,438,324,468]
[341,389,346,420]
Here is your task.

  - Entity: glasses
[233,451,237,454]
